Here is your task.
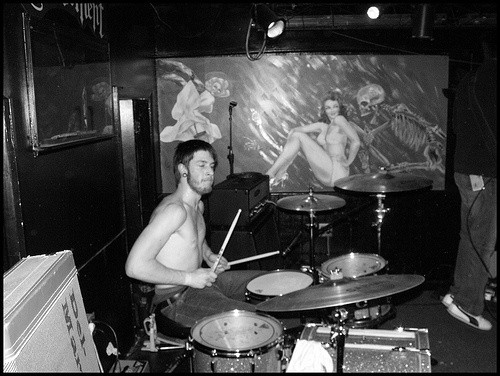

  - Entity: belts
[153,286,189,312]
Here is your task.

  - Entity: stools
[153,302,195,374]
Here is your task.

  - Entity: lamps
[367,4,384,19]
[411,2,434,39]
[249,2,285,38]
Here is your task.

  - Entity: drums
[316,250,391,326]
[242,267,314,343]
[187,308,285,373]
[284,322,434,373]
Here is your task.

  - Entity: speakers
[208,205,283,272]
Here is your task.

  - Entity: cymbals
[275,190,347,213]
[333,173,434,193]
[255,272,426,312]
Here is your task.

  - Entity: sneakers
[446,301,492,331]
[441,293,456,307]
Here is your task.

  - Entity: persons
[125,139,268,330]
[442,28,500,331]
